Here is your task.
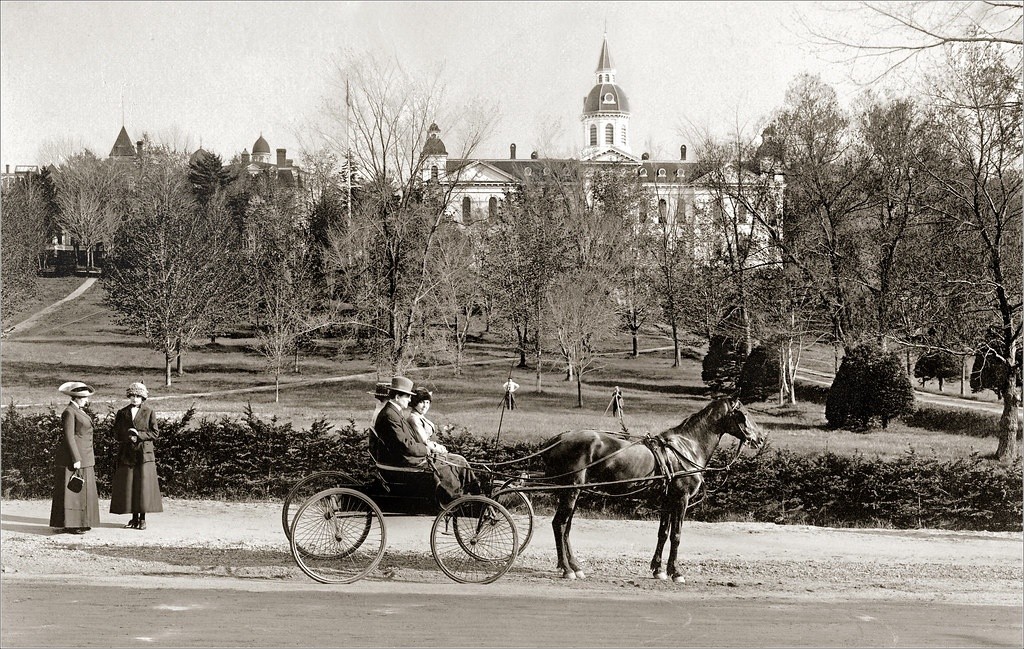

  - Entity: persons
[612,385,622,417]
[372,374,480,509]
[49,381,101,535]
[502,377,520,410]
[108,382,164,530]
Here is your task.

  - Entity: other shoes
[80,527,91,532]
[63,527,85,534]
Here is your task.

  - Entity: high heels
[125,520,138,529]
[139,519,146,530]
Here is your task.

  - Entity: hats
[365,383,392,397]
[389,376,418,395]
[408,386,431,407]
[125,382,148,401]
[58,381,96,397]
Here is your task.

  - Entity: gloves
[74,461,82,469]
[128,428,138,436]
[128,435,137,442]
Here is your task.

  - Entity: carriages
[282,389,765,587]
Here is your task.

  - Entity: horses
[540,386,765,584]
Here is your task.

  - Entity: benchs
[369,426,455,501]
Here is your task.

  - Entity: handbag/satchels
[66,469,84,494]
